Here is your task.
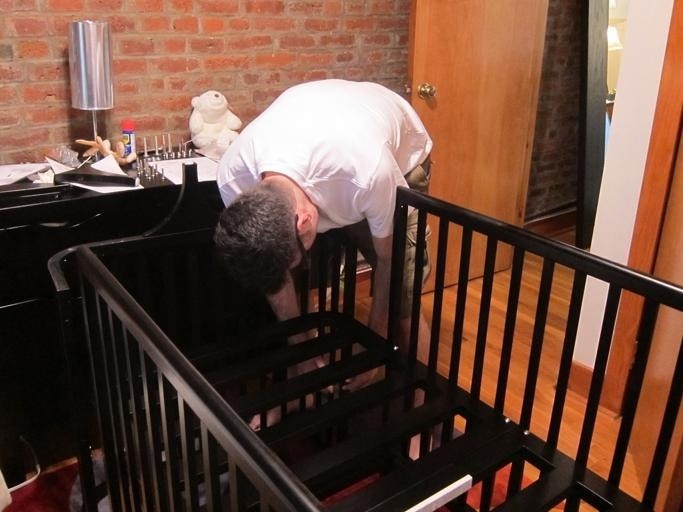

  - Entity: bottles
[121,119,136,158]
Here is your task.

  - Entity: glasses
[287,214,306,274]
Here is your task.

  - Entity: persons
[212,78,434,462]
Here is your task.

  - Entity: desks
[0,153,216,417]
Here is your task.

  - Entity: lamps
[69,21,116,167]
